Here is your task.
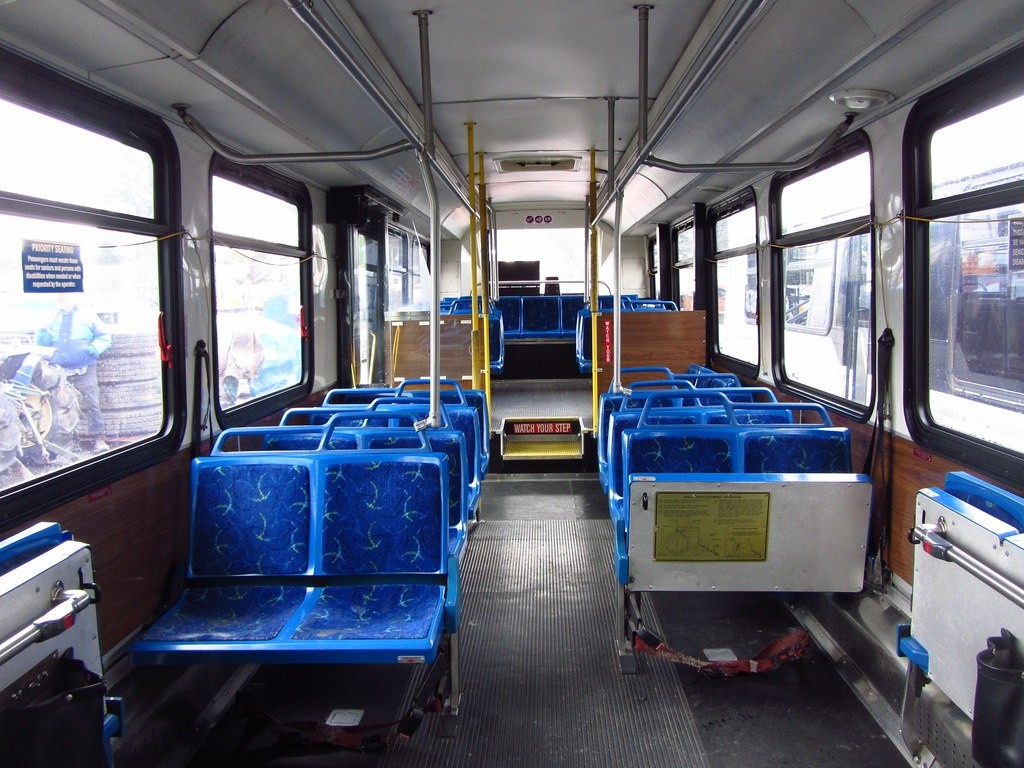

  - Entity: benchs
[598,367,874,676]
[131,378,490,714]
[436,294,680,375]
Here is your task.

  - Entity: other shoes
[62,440,81,451]
[93,440,110,452]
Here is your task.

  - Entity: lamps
[828,90,895,109]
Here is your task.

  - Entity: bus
[744,160,1024,455]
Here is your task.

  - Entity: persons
[36,293,112,454]
[216,288,265,409]
